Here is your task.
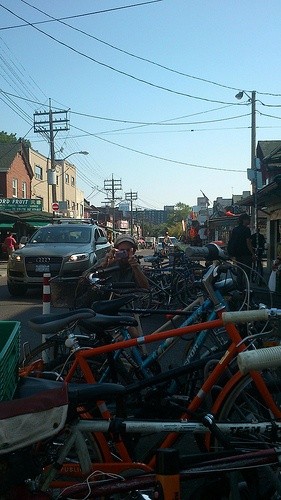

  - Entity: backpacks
[227,227,249,257]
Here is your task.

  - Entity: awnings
[25,221,50,228]
[0,222,15,231]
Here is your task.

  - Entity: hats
[113,234,137,249]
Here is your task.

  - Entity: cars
[153,236,177,256]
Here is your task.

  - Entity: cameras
[115,250,128,260]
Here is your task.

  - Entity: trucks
[144,237,156,250]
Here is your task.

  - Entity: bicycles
[0,240,281,500]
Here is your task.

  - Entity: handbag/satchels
[268,269,276,293]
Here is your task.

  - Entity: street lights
[61,150,90,199]
[234,89,258,234]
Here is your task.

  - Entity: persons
[20,234,27,245]
[4,234,16,255]
[251,228,266,259]
[230,214,256,304]
[162,234,172,254]
[82,234,149,354]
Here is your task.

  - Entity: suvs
[6,216,114,301]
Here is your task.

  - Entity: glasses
[119,248,132,253]
